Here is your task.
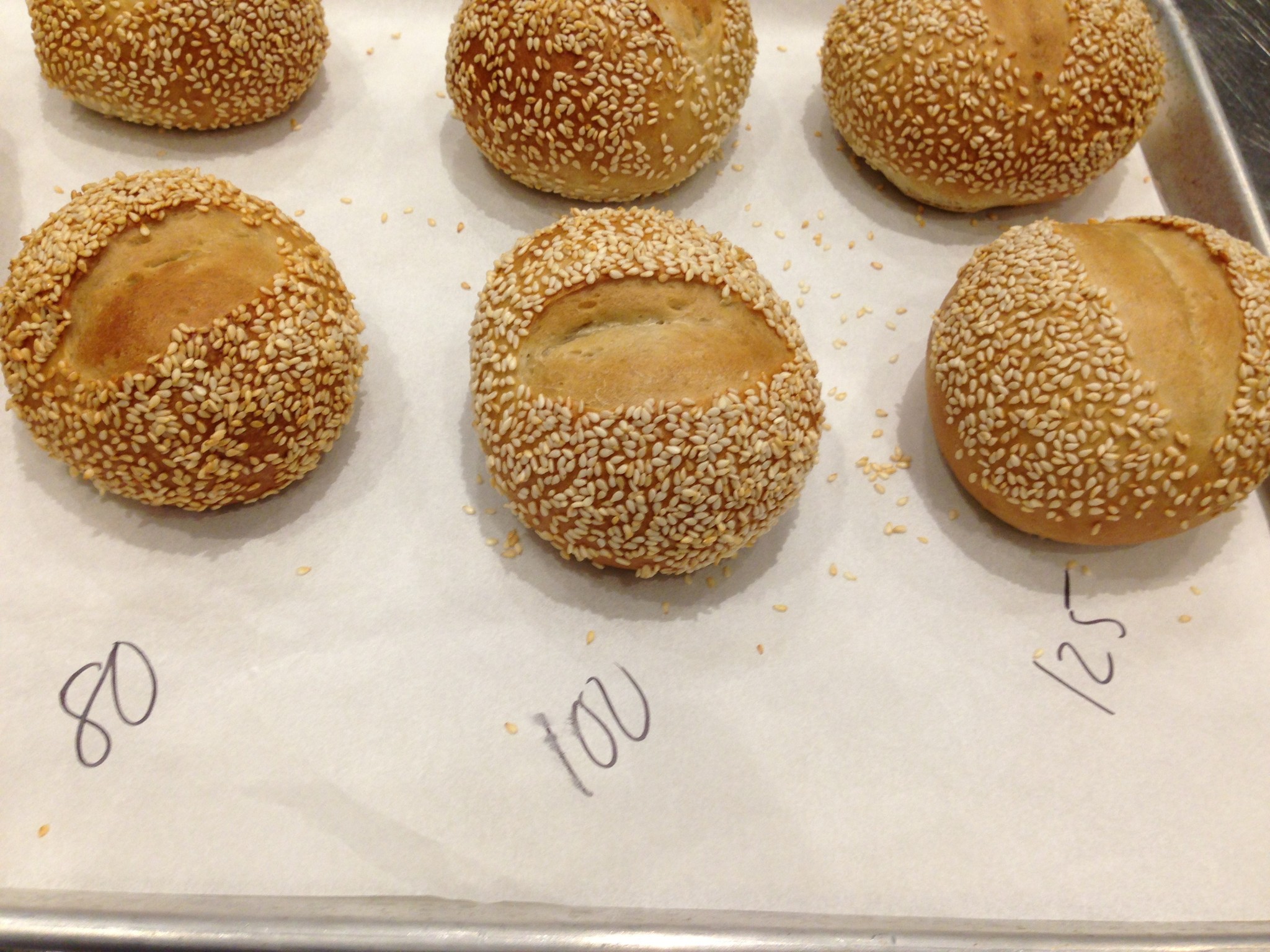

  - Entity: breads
[445,1,759,203]
[469,207,825,580]
[25,0,331,131]
[927,214,1270,545]
[820,1,1165,212]
[0,169,363,513]
